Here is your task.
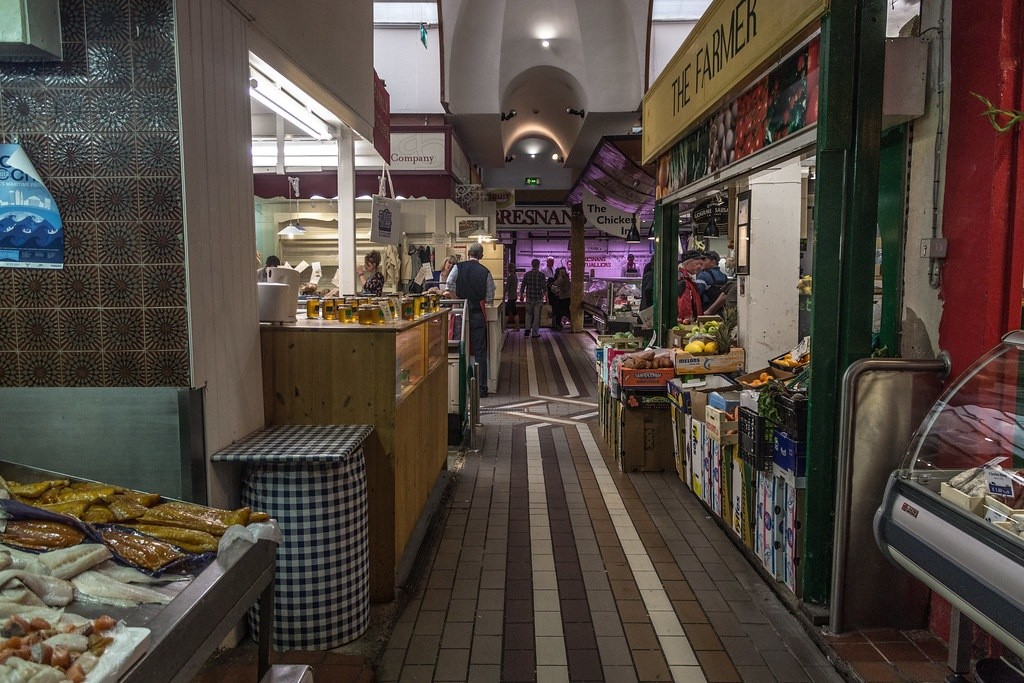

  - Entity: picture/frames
[455,215,489,242]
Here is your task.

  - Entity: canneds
[306,292,440,323]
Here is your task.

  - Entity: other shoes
[551,325,562,332]
[533,334,540,338]
[511,329,520,332]
[524,329,531,338]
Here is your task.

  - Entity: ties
[550,267,553,273]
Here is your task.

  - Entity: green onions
[668,124,708,194]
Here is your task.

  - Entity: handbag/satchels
[370,162,401,247]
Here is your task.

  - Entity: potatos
[624,351,673,369]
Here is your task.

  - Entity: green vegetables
[756,378,791,440]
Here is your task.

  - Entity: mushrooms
[707,100,738,176]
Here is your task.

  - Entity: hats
[702,251,720,261]
[681,250,705,261]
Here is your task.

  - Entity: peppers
[766,52,808,145]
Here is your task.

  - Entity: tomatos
[733,75,769,160]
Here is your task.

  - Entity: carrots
[724,406,739,435]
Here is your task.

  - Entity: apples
[671,319,722,337]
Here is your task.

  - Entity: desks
[211,424,375,652]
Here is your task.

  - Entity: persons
[257,256,280,282]
[677,250,738,321]
[519,259,548,338]
[540,256,572,330]
[445,243,496,398]
[504,263,521,332]
[356,250,384,298]
[638,254,654,325]
[426,255,458,290]
[621,254,642,277]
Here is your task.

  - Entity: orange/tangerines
[752,350,810,385]
[684,341,718,353]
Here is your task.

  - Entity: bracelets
[359,272,364,277]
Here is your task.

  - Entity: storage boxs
[940,482,1024,543]
[595,315,809,597]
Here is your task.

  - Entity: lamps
[506,155,515,162]
[552,154,564,163]
[566,107,584,118]
[455,184,498,244]
[626,213,640,244]
[703,205,719,238]
[277,176,305,238]
[501,109,517,121]
[648,222,655,240]
